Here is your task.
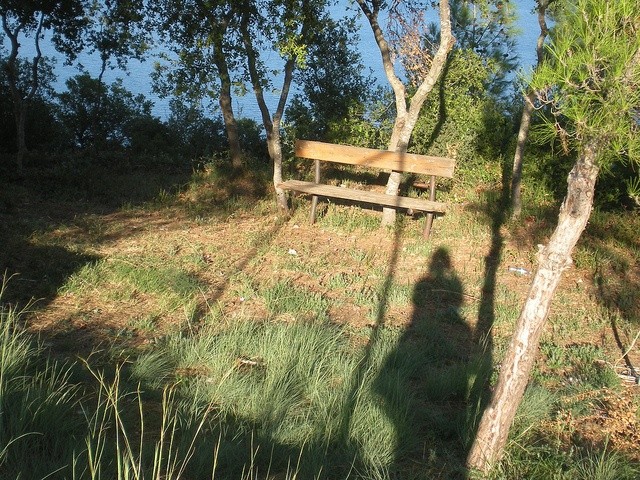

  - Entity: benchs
[275,139,455,241]
